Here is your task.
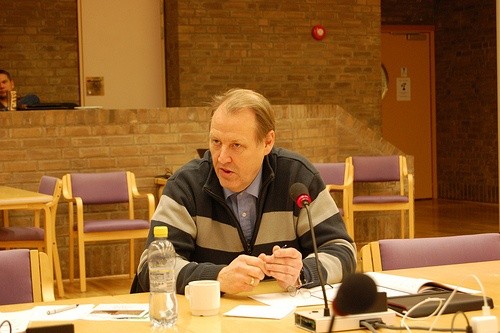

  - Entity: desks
[0,261,500,333]
[1,186,55,262]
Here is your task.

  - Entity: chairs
[62,170,156,293]
[0,174,66,306]
[311,154,500,273]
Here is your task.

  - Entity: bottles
[147,225,178,328]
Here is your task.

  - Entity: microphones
[288,183,330,316]
[328,272,378,332]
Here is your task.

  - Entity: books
[312,272,471,302]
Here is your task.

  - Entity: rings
[251,278,254,284]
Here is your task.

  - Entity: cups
[184,280,221,317]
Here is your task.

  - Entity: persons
[137,89,357,296]
[0,70,39,108]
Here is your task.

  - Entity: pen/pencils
[48,304,80,315]
